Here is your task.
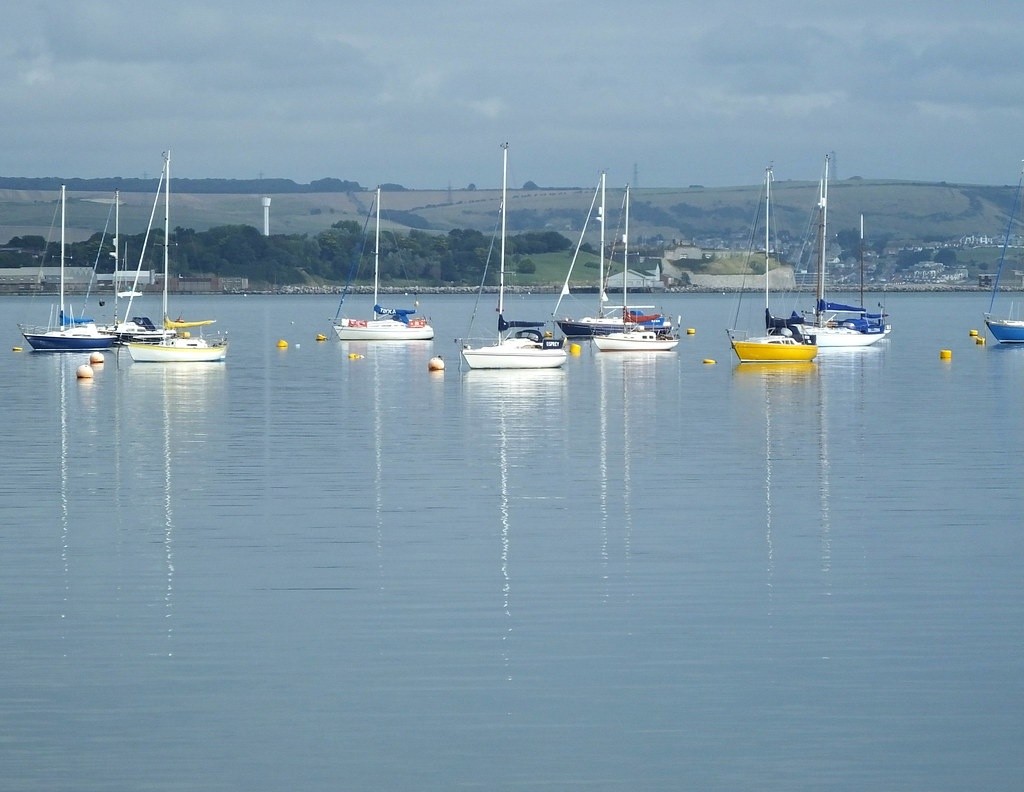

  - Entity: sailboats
[723,166,820,365]
[21,185,118,354]
[766,154,888,347]
[503,171,672,339]
[588,185,682,350]
[329,187,434,340]
[458,143,566,369]
[984,160,1024,343]
[124,150,230,363]
[18,184,176,343]
